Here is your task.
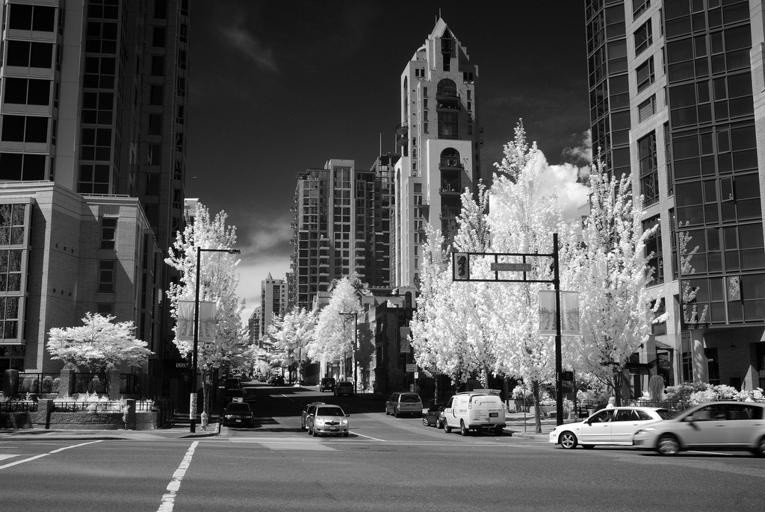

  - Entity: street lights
[189,246,240,433]
[339,312,357,394]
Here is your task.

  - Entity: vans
[439,392,506,436]
[223,378,242,396]
[385,391,423,418]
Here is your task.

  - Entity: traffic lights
[452,251,469,281]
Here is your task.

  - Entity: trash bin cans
[125,400,137,430]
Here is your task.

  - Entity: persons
[598,411,614,422]
[745,390,758,402]
[122,404,131,429]
[606,394,615,408]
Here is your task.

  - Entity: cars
[334,382,353,396]
[301,401,350,437]
[549,406,675,449]
[423,405,446,429]
[224,402,255,427]
[633,401,765,457]
[260,375,284,385]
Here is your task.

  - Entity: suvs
[320,378,335,392]
